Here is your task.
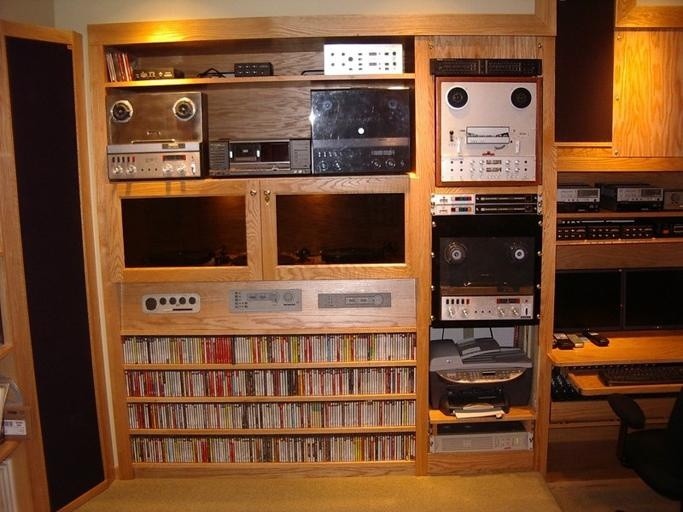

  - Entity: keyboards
[599,366,683,386]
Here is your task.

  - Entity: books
[122,333,416,463]
[106,50,137,82]
[0,374,20,512]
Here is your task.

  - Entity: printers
[429,338,533,419]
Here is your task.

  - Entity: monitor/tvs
[623,268,683,331]
[554,269,623,332]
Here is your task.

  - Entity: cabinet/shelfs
[86,0,683,476]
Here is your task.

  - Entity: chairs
[608,387,683,512]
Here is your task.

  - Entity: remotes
[553,337,558,348]
[585,332,608,346]
[567,334,584,347]
[553,333,573,349]
